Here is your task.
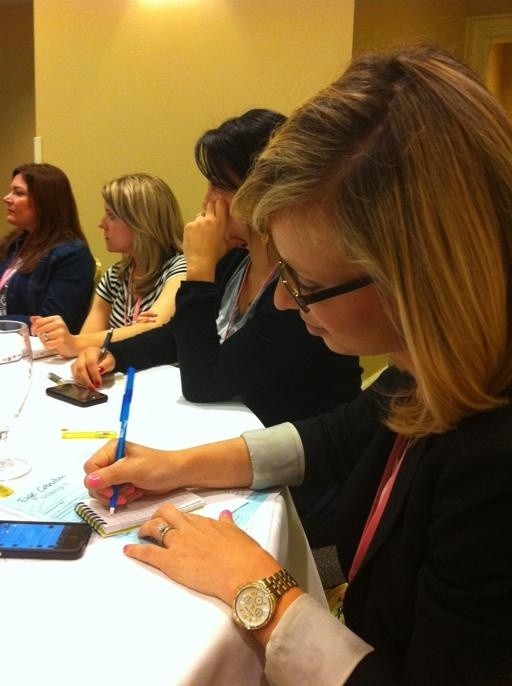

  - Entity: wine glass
[0,319,32,480]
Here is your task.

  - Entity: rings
[43,333,51,343]
[156,523,176,545]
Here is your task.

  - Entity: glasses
[278,258,379,314]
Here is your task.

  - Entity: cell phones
[46,383,108,407]
[0,520,93,561]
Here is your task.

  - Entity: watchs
[233,569,300,633]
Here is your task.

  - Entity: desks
[1,330,290,686]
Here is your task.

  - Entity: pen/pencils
[62,431,116,440]
[82,327,114,400]
[109,367,136,515]
[47,372,65,385]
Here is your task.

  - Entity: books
[76,489,207,540]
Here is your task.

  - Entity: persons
[29,174,188,360]
[83,38,512,686]
[0,165,94,335]
[72,108,361,548]
